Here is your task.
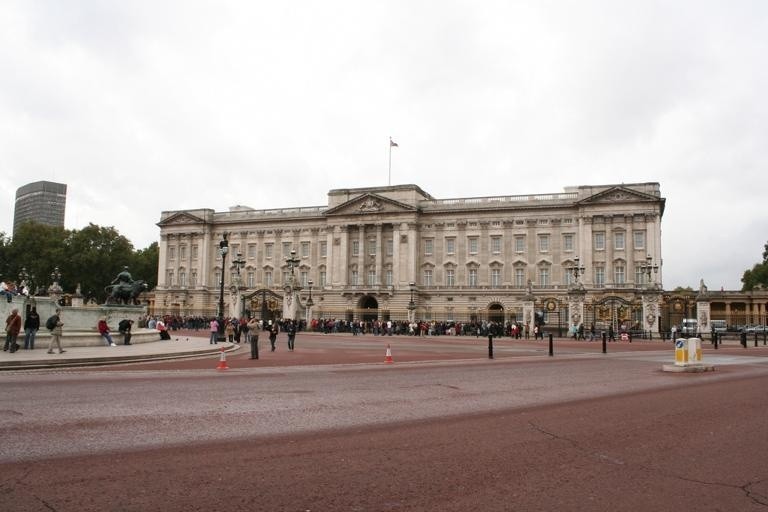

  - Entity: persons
[97,316,134,346]
[111,266,133,305]
[573,322,703,343]
[311,316,544,340]
[0,279,66,354]
[208,315,307,361]
[137,314,211,340]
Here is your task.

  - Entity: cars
[680,316,768,342]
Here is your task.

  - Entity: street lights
[307,280,313,302]
[17,266,29,281]
[408,282,415,302]
[233,251,244,275]
[142,281,150,303]
[640,254,659,284]
[50,265,60,284]
[212,232,232,344]
[526,278,533,295]
[568,256,584,282]
[285,250,299,275]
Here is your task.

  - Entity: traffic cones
[217,347,229,371]
[383,343,396,365]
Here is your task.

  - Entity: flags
[391,139,398,147]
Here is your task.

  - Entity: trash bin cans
[674,338,702,367]
